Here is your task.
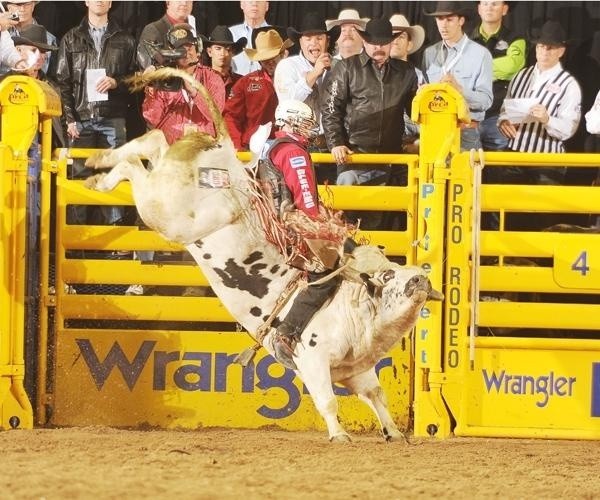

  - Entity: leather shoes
[272,333,297,369]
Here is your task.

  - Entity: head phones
[167,22,203,53]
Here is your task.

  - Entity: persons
[258,100,356,368]
[0,2,599,300]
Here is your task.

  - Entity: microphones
[188,53,202,66]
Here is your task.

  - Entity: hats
[166,9,424,62]
[424,2,474,20]
[530,20,576,47]
[12,24,60,51]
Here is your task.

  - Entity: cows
[83,66,446,446]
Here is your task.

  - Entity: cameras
[156,48,187,91]
[11,12,19,21]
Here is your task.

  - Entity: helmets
[274,99,317,140]
